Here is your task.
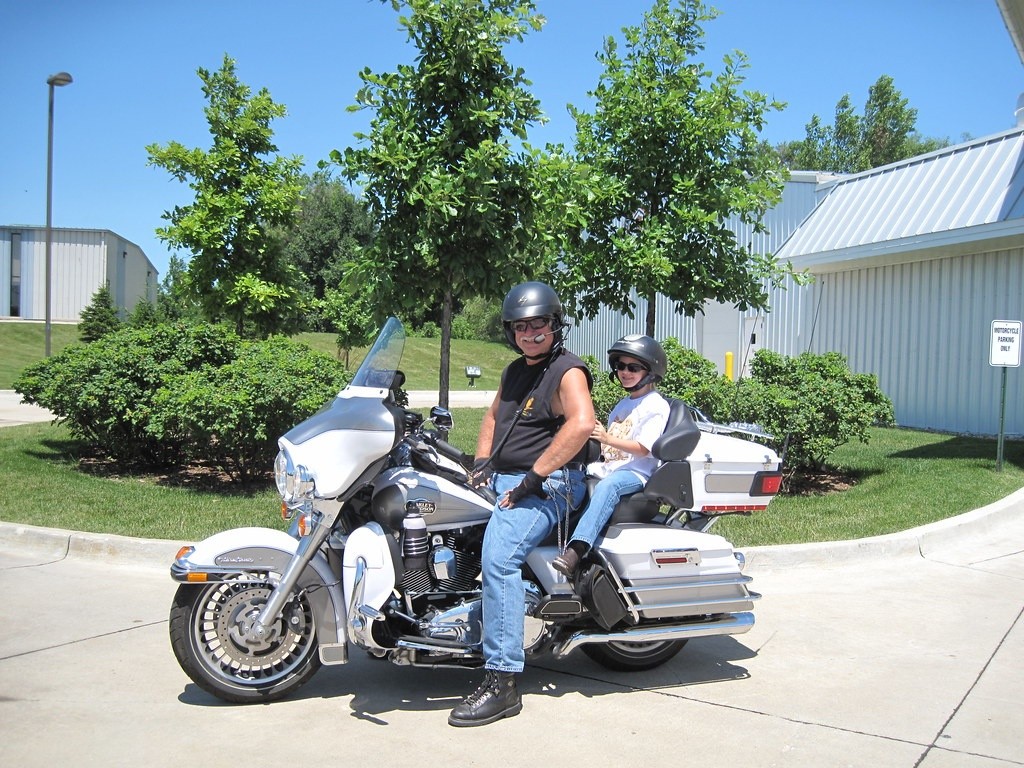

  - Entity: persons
[552,333,670,579]
[448,282,594,727]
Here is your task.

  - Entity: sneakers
[447,670,523,728]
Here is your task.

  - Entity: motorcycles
[169,316,783,710]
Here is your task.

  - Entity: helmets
[607,334,667,383]
[501,282,563,353]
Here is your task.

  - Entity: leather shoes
[551,546,583,579]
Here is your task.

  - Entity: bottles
[402,502,429,556]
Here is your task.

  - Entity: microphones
[534,320,566,344]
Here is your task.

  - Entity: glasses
[614,361,648,373]
[511,317,550,332]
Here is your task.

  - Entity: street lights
[44,71,74,357]
